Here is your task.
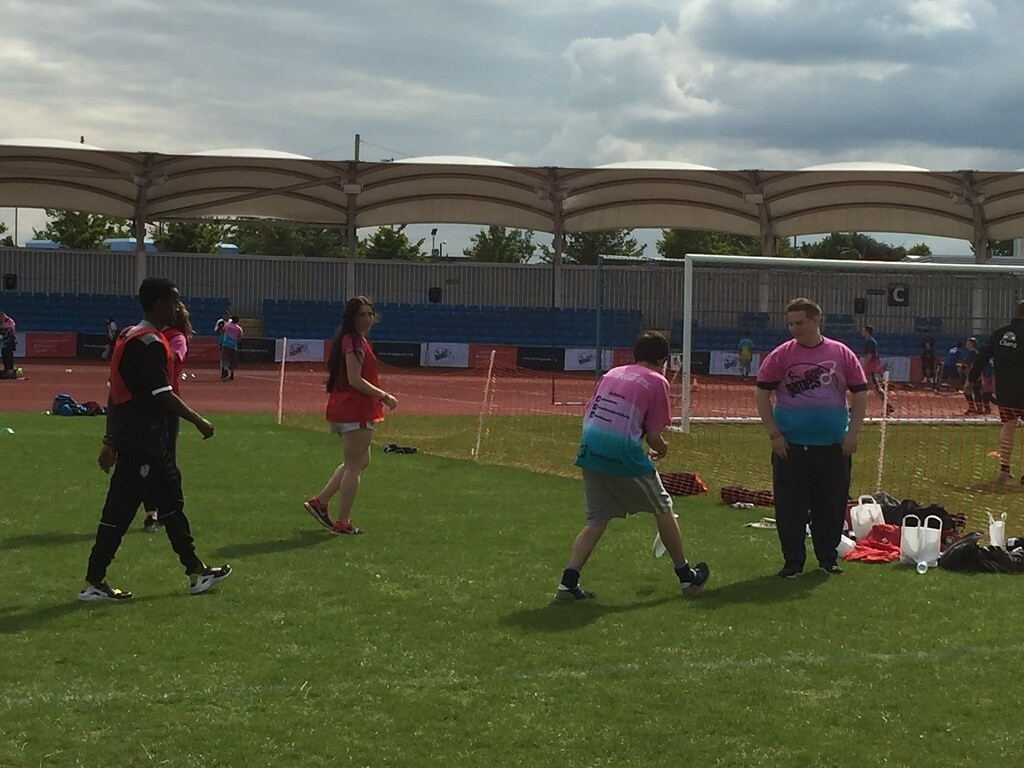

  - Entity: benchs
[263,299,643,349]
[671,313,987,356]
[5,293,230,336]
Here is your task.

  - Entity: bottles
[916,560,928,574]
[945,536,955,550]
[731,502,753,509]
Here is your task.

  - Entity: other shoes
[143,515,158,533]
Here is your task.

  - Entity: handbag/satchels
[986,511,1007,547]
[850,495,885,540]
[939,531,983,570]
[900,514,943,567]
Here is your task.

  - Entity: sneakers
[77,577,132,601]
[331,518,363,535]
[304,496,334,529]
[681,562,710,598]
[185,561,232,595]
[996,471,1017,485]
[778,561,804,578]
[819,560,844,575]
[555,584,597,599]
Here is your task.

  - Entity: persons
[78,277,232,600]
[861,325,895,416]
[302,296,399,535]
[0,311,15,370]
[552,330,711,602]
[920,335,997,416]
[965,300,1024,479]
[738,331,755,380]
[754,298,870,578]
[215,310,244,380]
[143,301,192,533]
[106,317,118,343]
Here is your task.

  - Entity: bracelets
[381,392,389,403]
[767,431,781,439]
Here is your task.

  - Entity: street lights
[439,241,447,257]
[431,228,438,249]
